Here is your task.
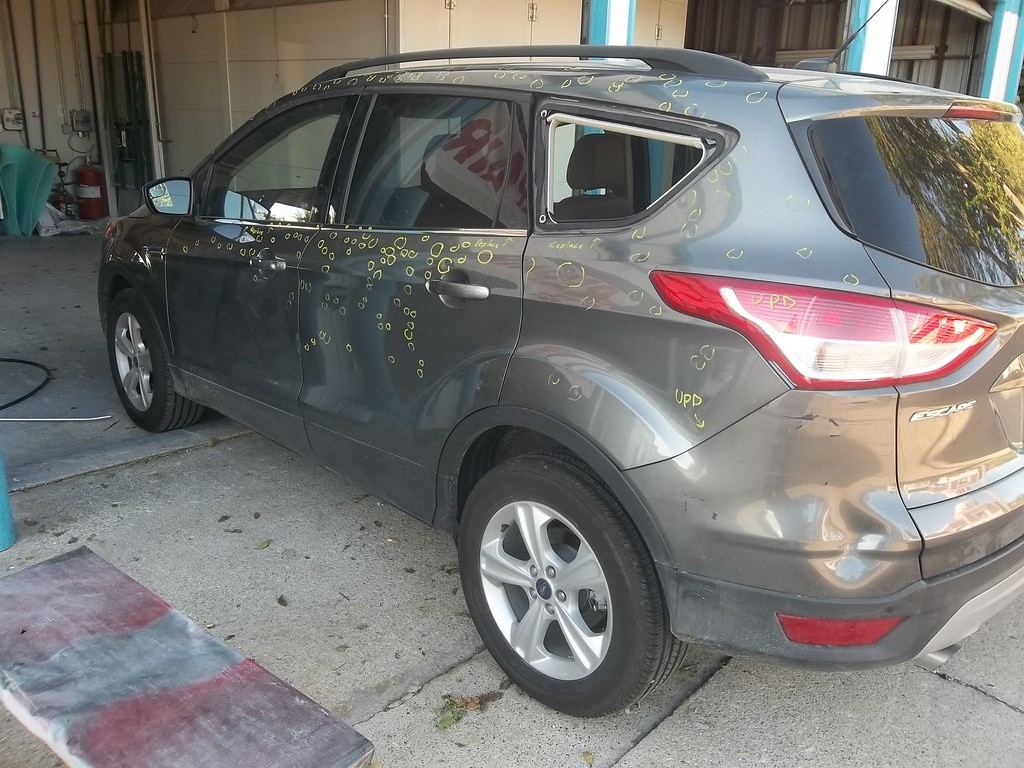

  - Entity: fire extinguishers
[76,153,108,218]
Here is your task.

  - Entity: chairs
[411,134,494,230]
[553,132,634,224]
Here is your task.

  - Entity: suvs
[98,41,1024,720]
